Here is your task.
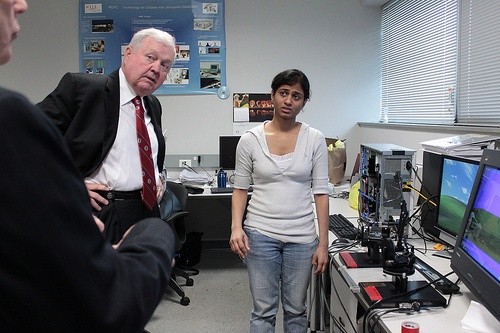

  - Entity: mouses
[331,238,353,248]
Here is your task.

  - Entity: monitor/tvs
[449,149,500,324]
[219,135,242,170]
[434,153,480,259]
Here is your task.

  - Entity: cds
[217,86,230,99]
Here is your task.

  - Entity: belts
[91,188,143,200]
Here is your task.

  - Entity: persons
[92,40,105,52]
[33,26,176,249]
[229,68,330,332]
[0,0,176,333]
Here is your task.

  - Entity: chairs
[166,180,194,306]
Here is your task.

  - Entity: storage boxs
[325,138,347,186]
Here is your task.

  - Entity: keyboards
[328,213,363,241]
[210,185,253,194]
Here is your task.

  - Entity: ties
[131,96,156,210]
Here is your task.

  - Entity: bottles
[218,168,225,188]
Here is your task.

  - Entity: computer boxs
[421,132,500,234]
[357,143,416,240]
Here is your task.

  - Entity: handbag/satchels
[325,138,346,186]
[158,186,182,220]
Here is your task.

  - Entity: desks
[166,176,254,265]
[304,176,500,333]
